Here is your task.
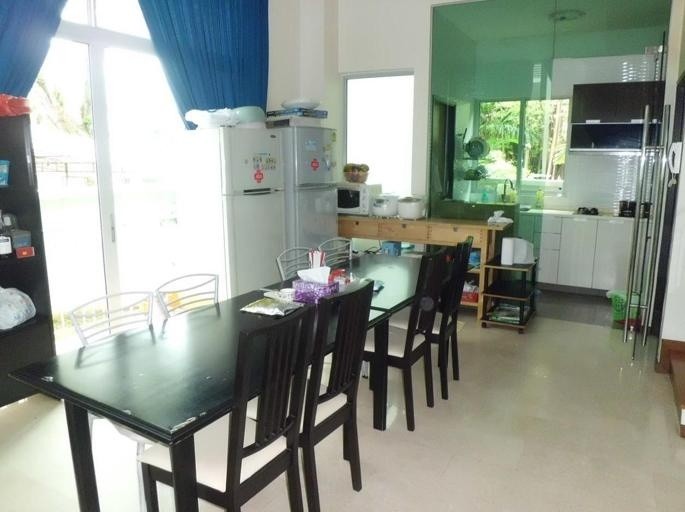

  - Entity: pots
[619,200,652,214]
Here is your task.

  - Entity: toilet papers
[501,236,535,266]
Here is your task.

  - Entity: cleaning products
[537,189,544,209]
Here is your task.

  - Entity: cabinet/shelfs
[557,214,648,298]
[480,255,537,334]
[527,210,562,292]
[0,114,59,411]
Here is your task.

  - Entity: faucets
[501,177,516,203]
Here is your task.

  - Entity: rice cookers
[372,194,427,220]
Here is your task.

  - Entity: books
[486,304,531,323]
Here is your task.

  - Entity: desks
[336,213,513,320]
[9,290,389,511]
[259,254,475,431]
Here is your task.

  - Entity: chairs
[277,247,312,280]
[66,291,155,457]
[357,246,448,430]
[139,302,317,512]
[390,235,473,398]
[317,237,353,266]
[154,273,220,316]
[248,279,375,512]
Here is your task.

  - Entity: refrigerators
[222,125,341,297]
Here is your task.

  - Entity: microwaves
[337,184,383,217]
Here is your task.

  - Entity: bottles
[535,188,544,209]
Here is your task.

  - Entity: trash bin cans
[611,289,640,330]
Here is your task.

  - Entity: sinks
[519,206,531,212]
